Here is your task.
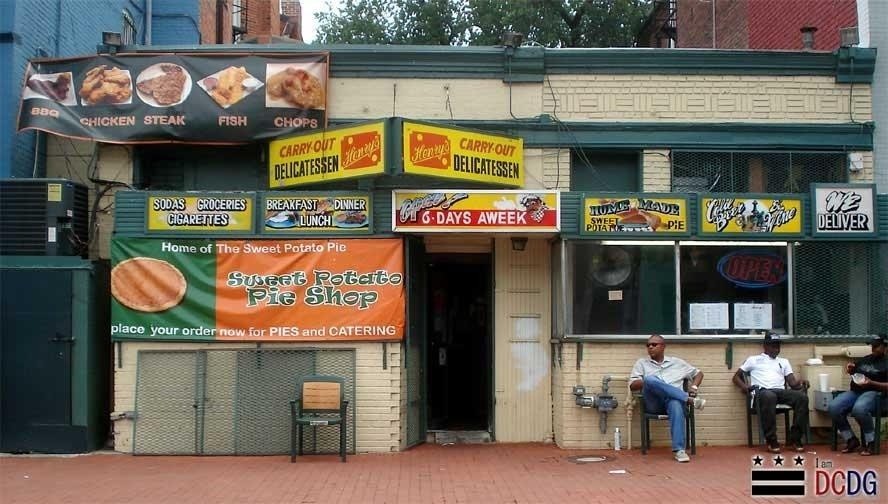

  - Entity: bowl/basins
[852,373,866,385]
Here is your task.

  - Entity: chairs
[832,390,888,454]
[742,360,812,448]
[637,379,695,454]
[290,376,350,462]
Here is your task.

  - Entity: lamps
[103,31,122,46]
[840,27,863,46]
[503,32,523,49]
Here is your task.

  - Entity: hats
[764,331,781,344]
[865,333,888,347]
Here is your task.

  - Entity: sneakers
[692,398,707,410]
[792,439,804,451]
[859,441,874,456]
[841,437,861,453]
[767,442,781,453]
[673,449,690,462]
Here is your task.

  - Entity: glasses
[646,341,663,347]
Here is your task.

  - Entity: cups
[818,373,832,392]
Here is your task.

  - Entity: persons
[829,334,888,456]
[629,335,706,462]
[732,332,810,453]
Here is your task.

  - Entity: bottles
[613,428,622,450]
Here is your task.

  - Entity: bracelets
[690,385,698,391]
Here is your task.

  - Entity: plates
[23,63,326,112]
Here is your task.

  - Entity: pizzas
[111,257,187,312]
